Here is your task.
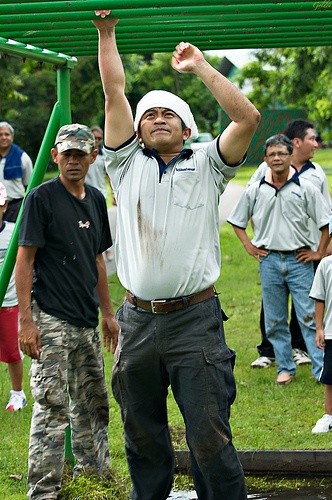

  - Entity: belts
[127,286,216,314]
[261,246,308,255]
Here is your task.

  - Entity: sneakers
[292,348,312,366]
[251,357,271,368]
[312,414,332,433]
[5,393,27,411]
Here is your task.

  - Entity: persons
[15,124,119,500]
[92,7,260,500]
[87,126,109,199]
[311,254,332,433]
[0,121,33,411]
[227,120,332,383]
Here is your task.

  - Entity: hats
[0,182,7,206]
[53,123,95,153]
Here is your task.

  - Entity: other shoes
[277,372,294,384]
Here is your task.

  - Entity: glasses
[267,151,290,158]
[94,137,102,141]
